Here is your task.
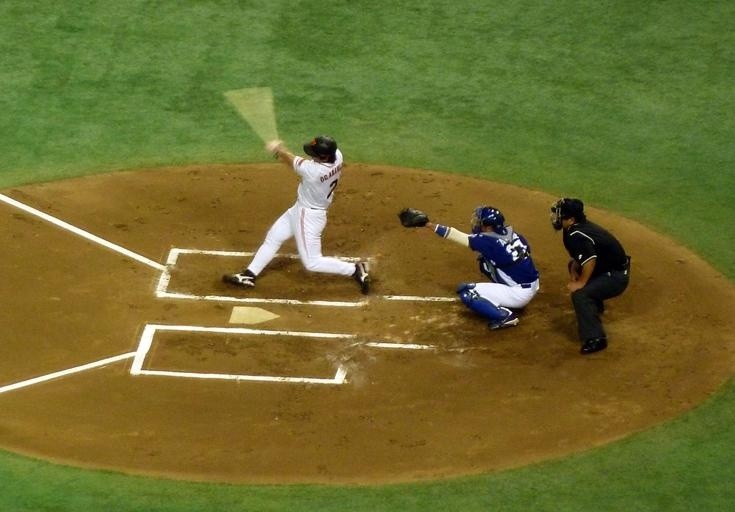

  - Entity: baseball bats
[223,86,278,146]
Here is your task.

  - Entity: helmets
[470,206,508,235]
[303,136,337,158]
[550,198,588,230]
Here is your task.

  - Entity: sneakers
[488,311,519,330]
[353,262,370,293]
[223,271,256,289]
[580,337,607,354]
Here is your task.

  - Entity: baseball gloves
[398,207,428,227]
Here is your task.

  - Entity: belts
[522,284,531,288]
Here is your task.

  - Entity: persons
[222,134,370,295]
[550,198,630,355]
[397,205,541,330]
